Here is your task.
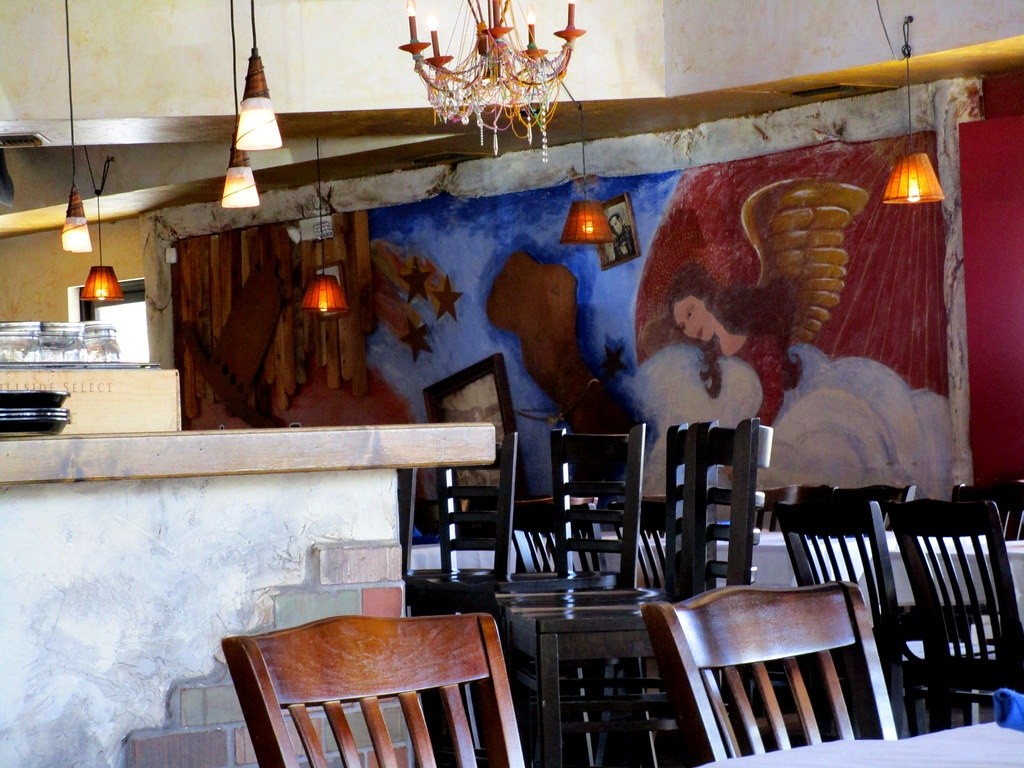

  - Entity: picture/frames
[595,191,642,271]
[420,352,528,514]
[313,260,346,295]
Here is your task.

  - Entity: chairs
[639,581,900,766]
[507,417,775,768]
[824,484,918,533]
[756,483,837,531]
[515,493,600,532]
[501,419,721,748]
[219,610,525,768]
[637,494,666,535]
[407,425,522,618]
[779,497,971,737]
[887,499,1024,733]
[416,422,648,748]
[907,479,1024,681]
[483,422,690,717]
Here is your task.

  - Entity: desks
[572,530,898,588]
[453,528,572,581]
[700,721,1024,768]
[410,543,442,571]
[855,539,1024,659]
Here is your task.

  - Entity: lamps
[235,0,283,149]
[557,102,616,243]
[78,189,125,302]
[221,0,261,209]
[398,0,587,163]
[881,17,946,203]
[61,0,93,253]
[302,137,350,313]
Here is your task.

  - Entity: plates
[0,408,72,437]
[0,389,71,408]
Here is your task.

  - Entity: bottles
[80,320,121,363]
[0,321,42,363]
[41,322,89,363]
